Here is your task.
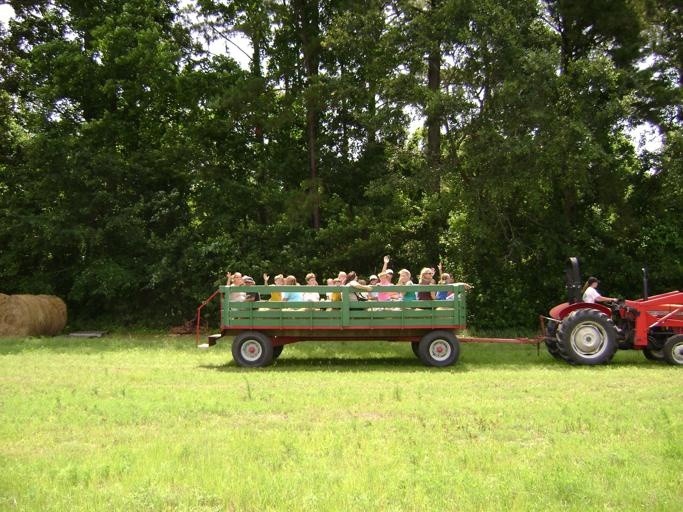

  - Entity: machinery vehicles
[541,254,682,366]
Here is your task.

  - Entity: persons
[224,256,471,319]
[582,277,622,332]
